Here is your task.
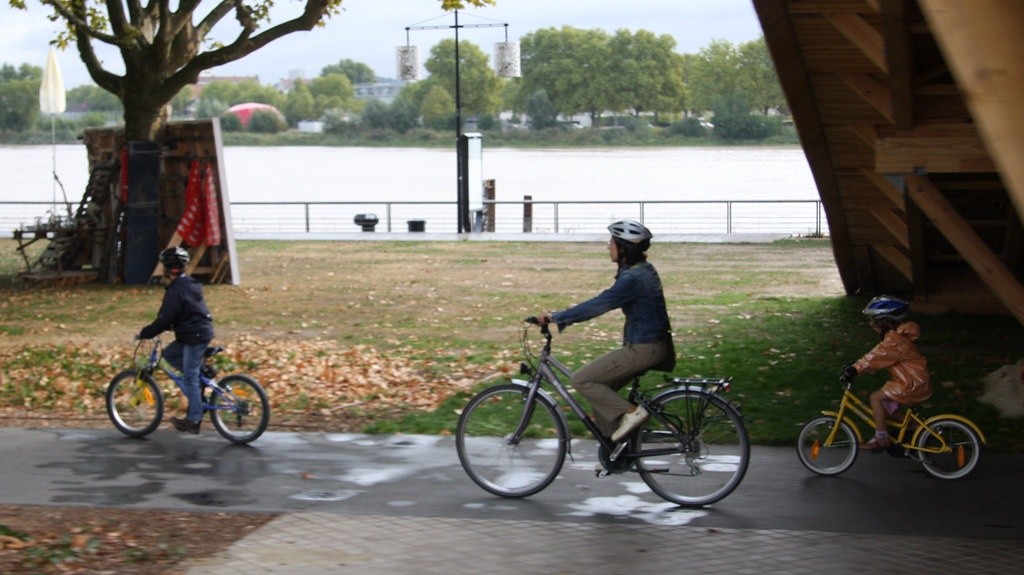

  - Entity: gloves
[843,366,856,384]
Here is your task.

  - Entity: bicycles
[455,317,751,506]
[105,336,270,443]
[797,363,988,481]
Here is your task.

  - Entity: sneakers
[611,404,650,442]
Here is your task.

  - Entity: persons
[133,245,216,434]
[842,294,937,449]
[541,218,679,472]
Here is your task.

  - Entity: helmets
[861,295,910,321]
[607,222,652,251]
[160,246,190,269]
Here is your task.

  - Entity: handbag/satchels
[651,332,676,372]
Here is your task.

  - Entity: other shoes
[596,464,605,470]
[859,436,891,448]
[171,417,202,434]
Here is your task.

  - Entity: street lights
[396,10,522,233]
